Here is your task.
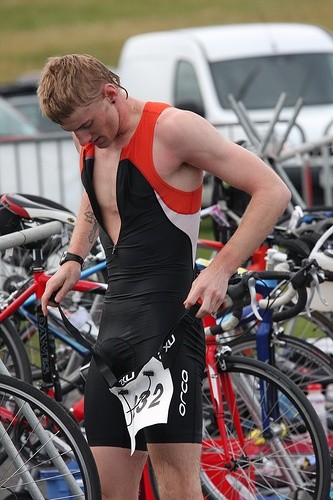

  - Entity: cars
[0,81,69,139]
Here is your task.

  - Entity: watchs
[59,251,85,271]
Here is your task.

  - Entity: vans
[117,23,333,207]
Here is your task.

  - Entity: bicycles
[0,189,332,500]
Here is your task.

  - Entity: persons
[36,53,291,500]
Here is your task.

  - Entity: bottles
[305,384,329,441]
[66,302,98,336]
